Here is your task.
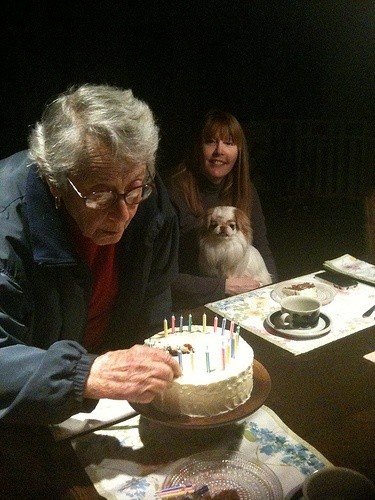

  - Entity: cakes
[142,325,255,418]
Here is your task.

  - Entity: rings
[258,281,263,288]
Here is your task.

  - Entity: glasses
[65,167,157,211]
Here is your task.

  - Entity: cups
[278,295,322,331]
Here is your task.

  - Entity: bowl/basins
[303,467,375,500]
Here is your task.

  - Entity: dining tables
[0,256,375,500]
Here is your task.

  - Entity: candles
[163,313,241,374]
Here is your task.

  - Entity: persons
[162,110,278,312]
[0,82,179,500]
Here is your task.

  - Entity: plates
[126,359,272,430]
[273,280,337,306]
[265,309,333,337]
[284,481,304,500]
[160,449,284,500]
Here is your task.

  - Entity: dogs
[198,206,272,289]
